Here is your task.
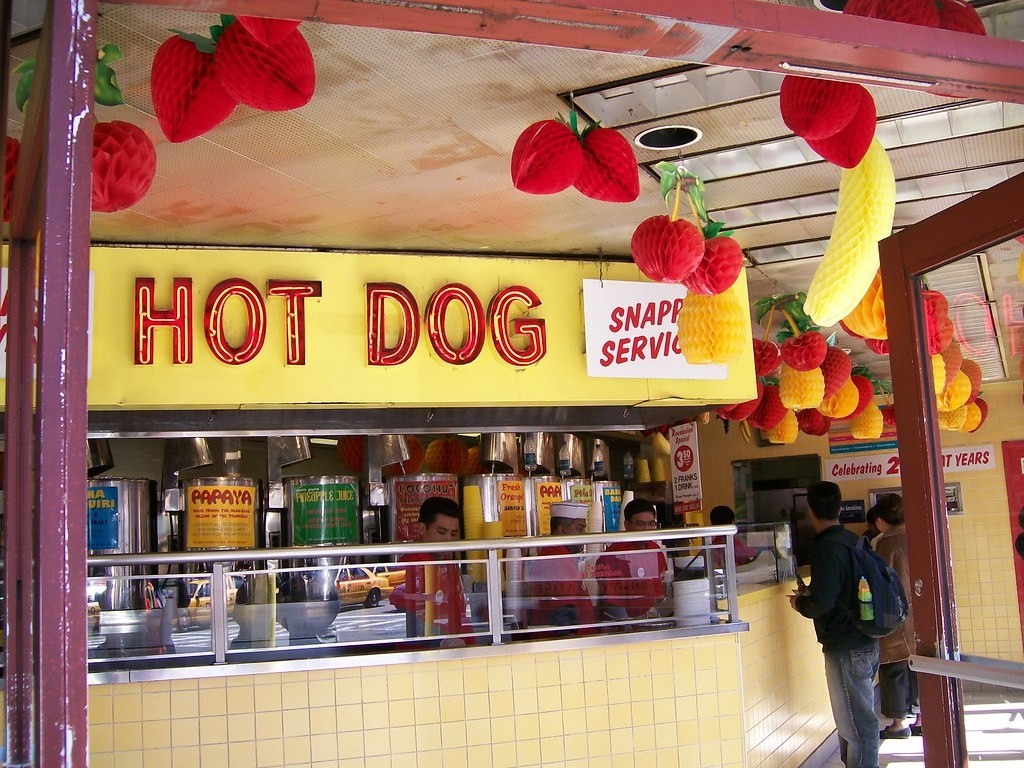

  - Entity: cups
[506,548,523,623]
[463,486,505,592]
[619,491,634,531]
[586,500,603,607]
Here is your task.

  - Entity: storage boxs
[708,521,795,582]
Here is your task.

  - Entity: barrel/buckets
[282,475,360,602]
[85,477,157,611]
[469,473,622,537]
[178,477,259,607]
[389,473,460,562]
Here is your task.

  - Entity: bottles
[858,576,874,620]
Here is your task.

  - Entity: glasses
[627,520,656,528]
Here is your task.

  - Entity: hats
[550,502,588,519]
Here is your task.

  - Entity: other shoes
[880,726,911,739]
[909,724,922,735]
[906,710,916,717]
[912,703,919,708]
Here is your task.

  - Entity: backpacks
[817,530,908,638]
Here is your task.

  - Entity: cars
[173,578,211,631]
[389,579,422,613]
[327,567,395,608]
[224,574,245,617]
[85,583,109,632]
[368,566,406,588]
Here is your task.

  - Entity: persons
[790,480,881,768]
[228,557,340,650]
[387,497,476,650]
[861,493,922,739]
[594,499,669,619]
[710,506,757,569]
[529,501,597,637]
